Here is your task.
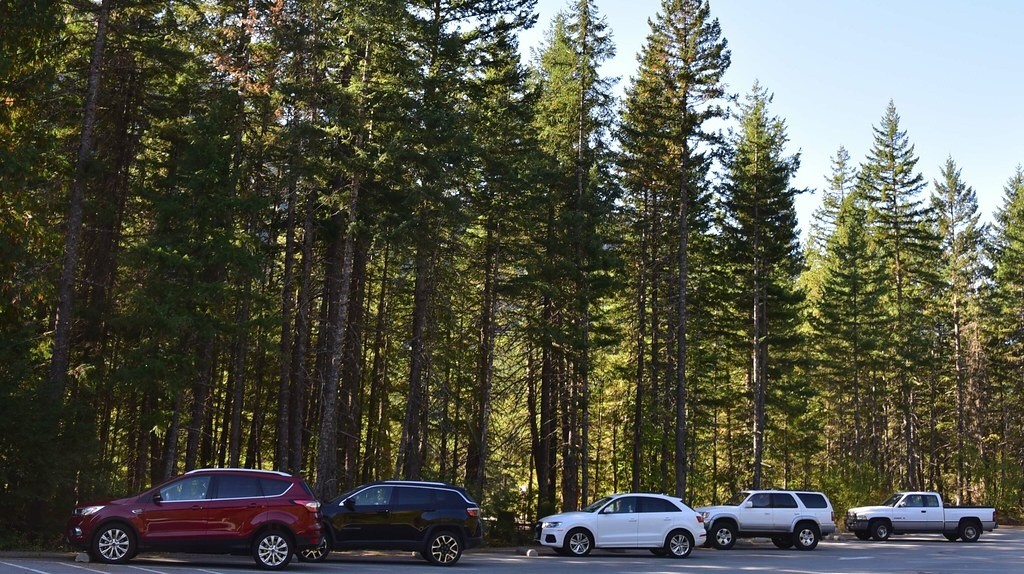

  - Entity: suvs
[533,492,707,559]
[294,480,484,567]
[693,490,836,551]
[67,468,324,571]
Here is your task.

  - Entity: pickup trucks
[844,491,997,542]
